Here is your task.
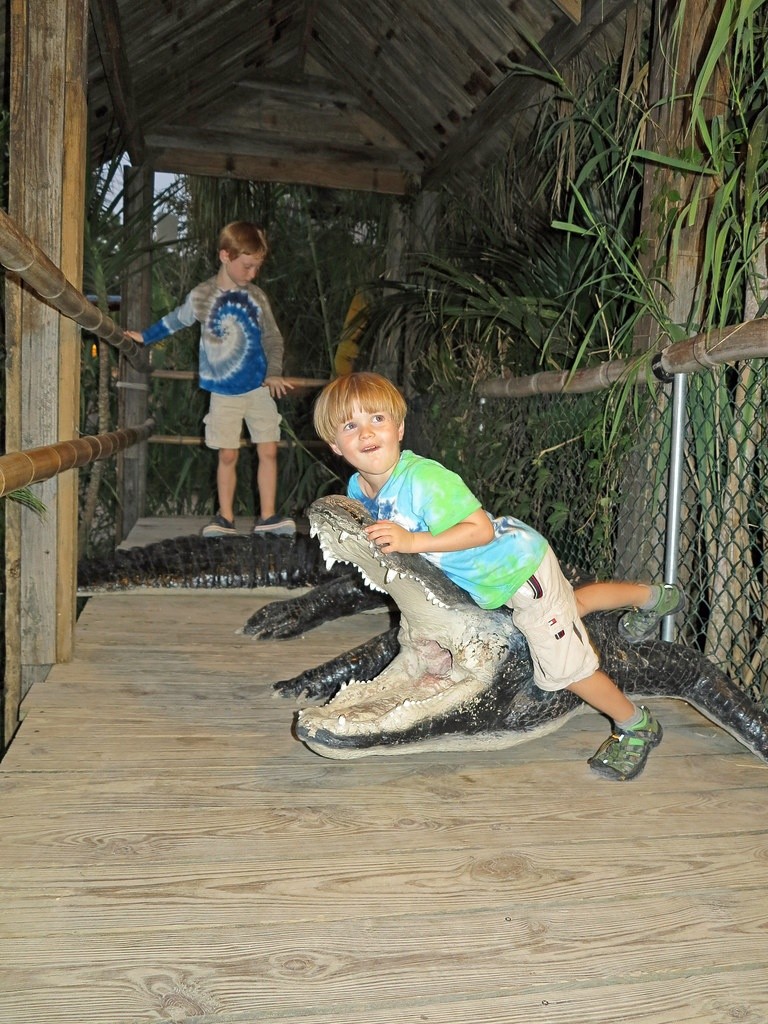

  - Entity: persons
[123,221,295,534]
[315,373,686,781]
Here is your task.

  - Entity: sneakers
[605,582,685,644]
[586,705,663,783]
[203,515,237,537]
[254,515,296,532]
